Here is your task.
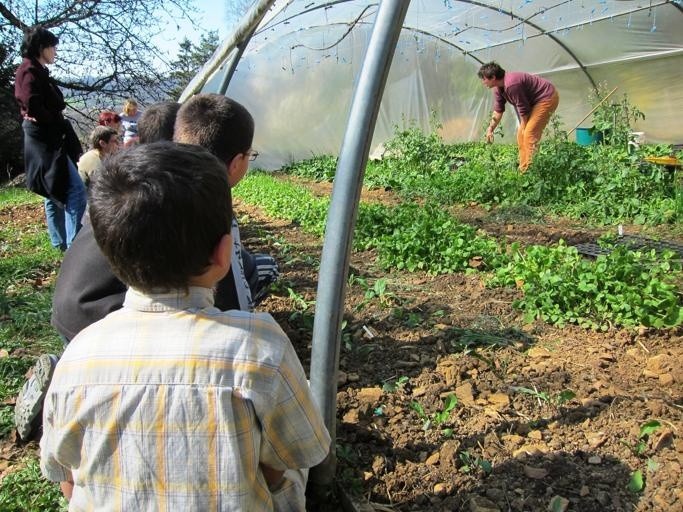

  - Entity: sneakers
[14,352,58,442]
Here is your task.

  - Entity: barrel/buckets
[576,127,602,146]
[629,132,645,150]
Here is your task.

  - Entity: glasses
[245,150,259,162]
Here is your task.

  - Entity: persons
[12,92,256,445]
[135,101,278,308]
[76,126,122,216]
[96,110,140,152]
[13,26,86,253]
[34,141,333,511]
[115,98,145,147]
[477,60,561,172]
